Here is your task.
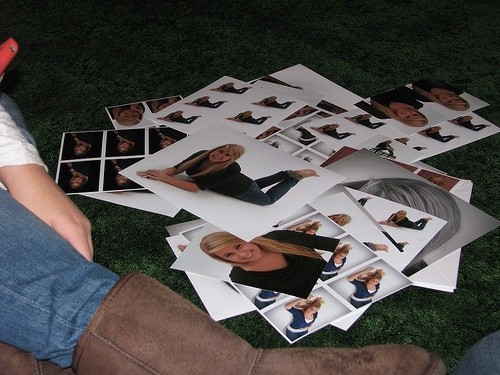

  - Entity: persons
[56,68,492,344]
[0,91,447,375]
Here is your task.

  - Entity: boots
[0,340,76,375]
[72,272,445,375]
